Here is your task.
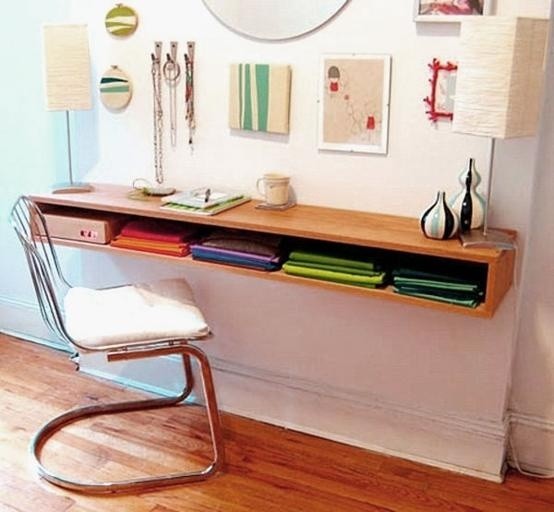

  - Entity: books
[162,184,243,208]
[164,197,252,217]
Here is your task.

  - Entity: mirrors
[202,0,349,45]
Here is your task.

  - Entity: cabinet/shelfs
[30,187,518,321]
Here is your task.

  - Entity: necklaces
[150,59,195,184]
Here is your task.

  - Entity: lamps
[42,23,94,194]
[451,15,551,251]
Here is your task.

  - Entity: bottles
[449,158,486,231]
[420,190,460,241]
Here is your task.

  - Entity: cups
[254,174,292,204]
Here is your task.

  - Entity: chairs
[7,196,224,497]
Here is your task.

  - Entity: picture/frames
[412,0,493,25]
[422,57,457,124]
[319,54,392,154]
